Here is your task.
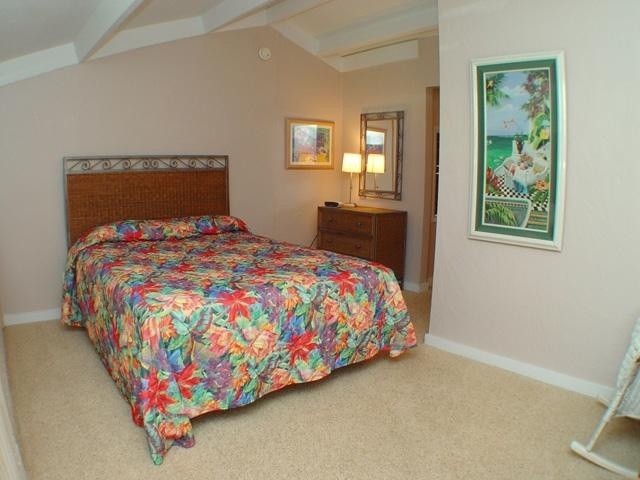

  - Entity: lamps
[342,152,362,208]
[366,154,386,190]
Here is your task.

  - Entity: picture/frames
[468,50,566,252]
[366,127,385,155]
[284,118,339,171]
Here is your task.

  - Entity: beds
[64,155,398,419]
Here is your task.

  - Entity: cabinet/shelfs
[318,205,407,292]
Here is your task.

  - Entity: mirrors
[358,111,404,201]
